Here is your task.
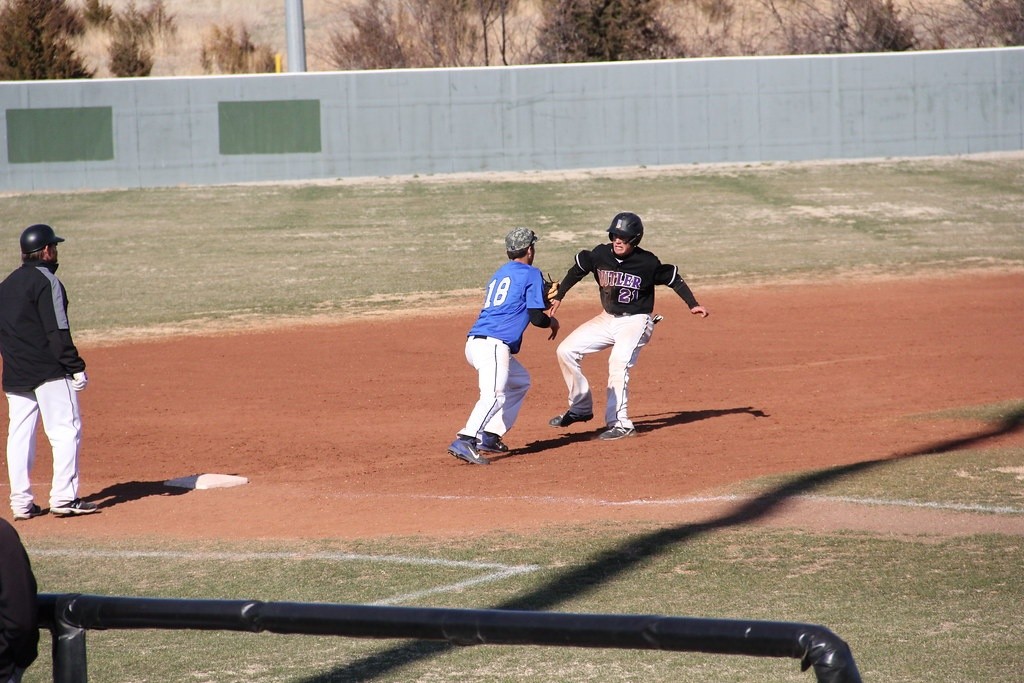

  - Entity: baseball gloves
[542,273,558,311]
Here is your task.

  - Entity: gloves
[71,371,88,392]
[652,315,664,324]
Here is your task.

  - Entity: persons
[448,227,559,463]
[0,224,98,520]
[550,212,709,440]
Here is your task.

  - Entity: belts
[474,335,509,345]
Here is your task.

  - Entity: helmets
[19,223,65,254]
[606,212,644,247]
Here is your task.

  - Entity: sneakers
[549,409,594,427]
[49,498,97,514]
[600,426,637,441]
[475,432,508,453]
[447,437,490,465]
[14,502,41,520]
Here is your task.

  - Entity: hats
[505,227,539,251]
